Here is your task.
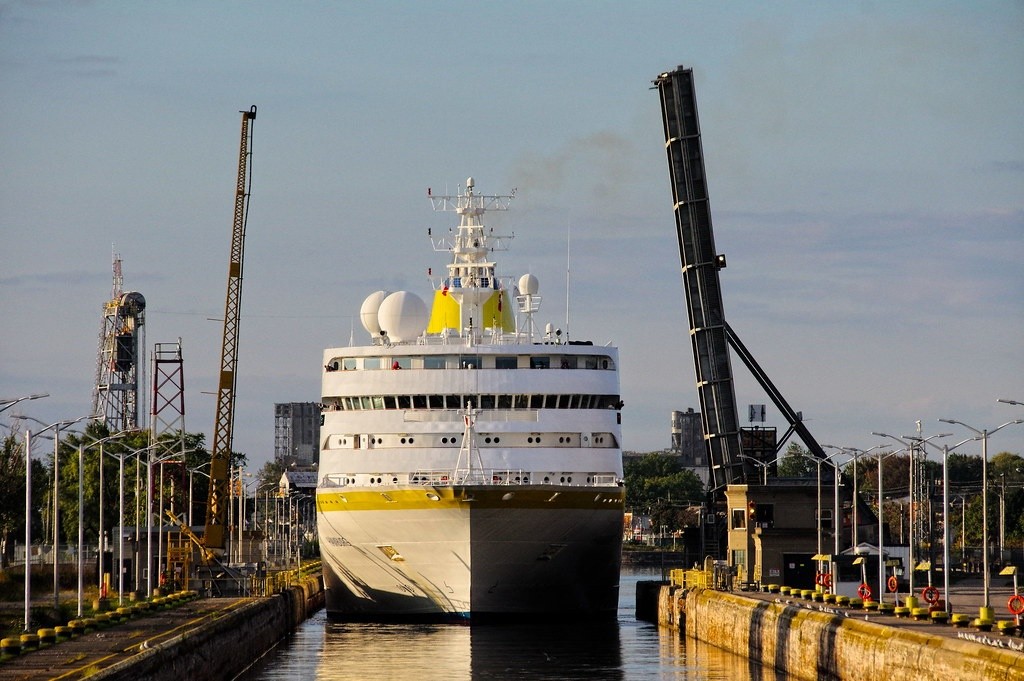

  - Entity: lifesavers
[923,586,939,603]
[1008,594,1024,614]
[857,584,872,599]
[816,573,832,586]
[888,577,898,592]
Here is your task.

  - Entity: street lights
[900,435,991,614]
[7,413,104,608]
[819,444,892,554]
[62,429,141,600]
[737,455,795,485]
[871,432,954,594]
[232,474,312,567]
[785,450,846,554]
[937,419,1024,606]
[37,434,127,617]
[1,419,83,632]
[107,439,175,591]
[89,446,161,605]
[841,447,920,606]
[114,454,181,598]
[810,455,860,554]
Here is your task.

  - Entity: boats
[314,177,626,626]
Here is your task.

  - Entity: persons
[333,401,340,410]
[392,361,401,370]
[324,364,334,371]
